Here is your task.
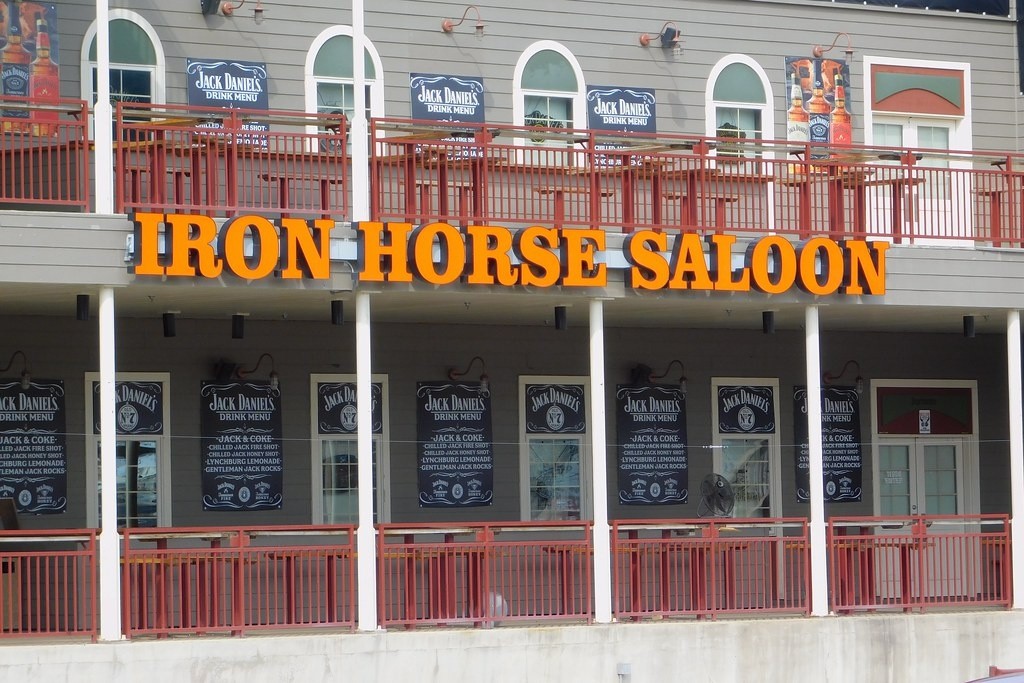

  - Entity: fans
[697,474,735,518]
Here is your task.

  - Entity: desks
[136,116,194,214]
[991,161,1024,182]
[617,145,685,234]
[386,131,457,224]
[428,156,507,224]
[798,168,876,241]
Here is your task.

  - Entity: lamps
[647,359,688,393]
[236,352,279,390]
[813,32,857,68]
[823,360,864,393]
[0,351,32,389]
[441,5,489,42]
[449,356,489,393]
[640,21,686,62]
[222,0,269,25]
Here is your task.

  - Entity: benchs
[969,185,1024,248]
[567,166,648,230]
[533,186,615,230]
[844,177,926,245]
[257,172,348,221]
[369,151,420,222]
[399,179,482,228]
[635,169,721,234]
[777,174,866,241]
[91,141,255,217]
[7,539,1012,642]
[664,192,739,235]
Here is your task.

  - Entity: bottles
[786,60,852,175]
[0,4,59,137]
